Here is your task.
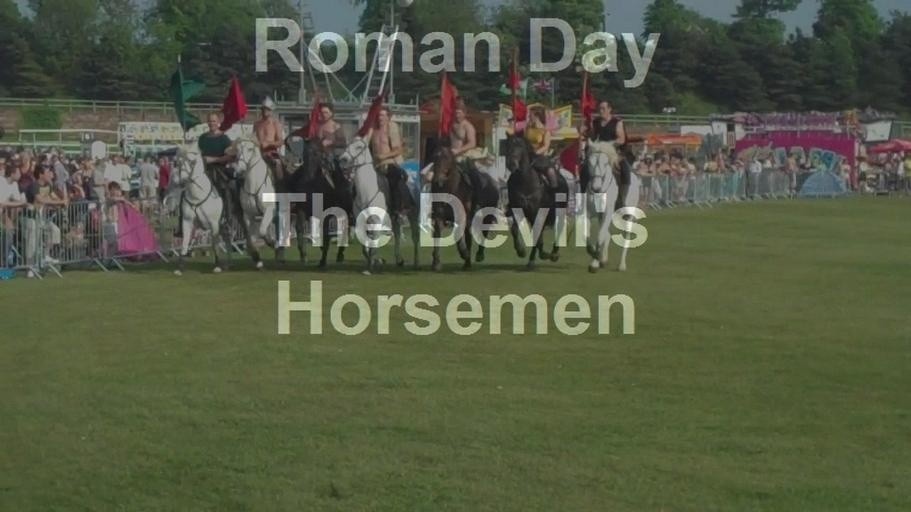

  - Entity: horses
[585,138,641,273]
[431,136,500,272]
[337,126,420,277]
[504,129,570,269]
[172,141,229,276]
[289,134,355,273]
[229,131,296,269]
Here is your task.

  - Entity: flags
[164,60,599,137]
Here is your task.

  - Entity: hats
[455,97,467,113]
[259,96,276,110]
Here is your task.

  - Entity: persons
[0,99,910,267]
